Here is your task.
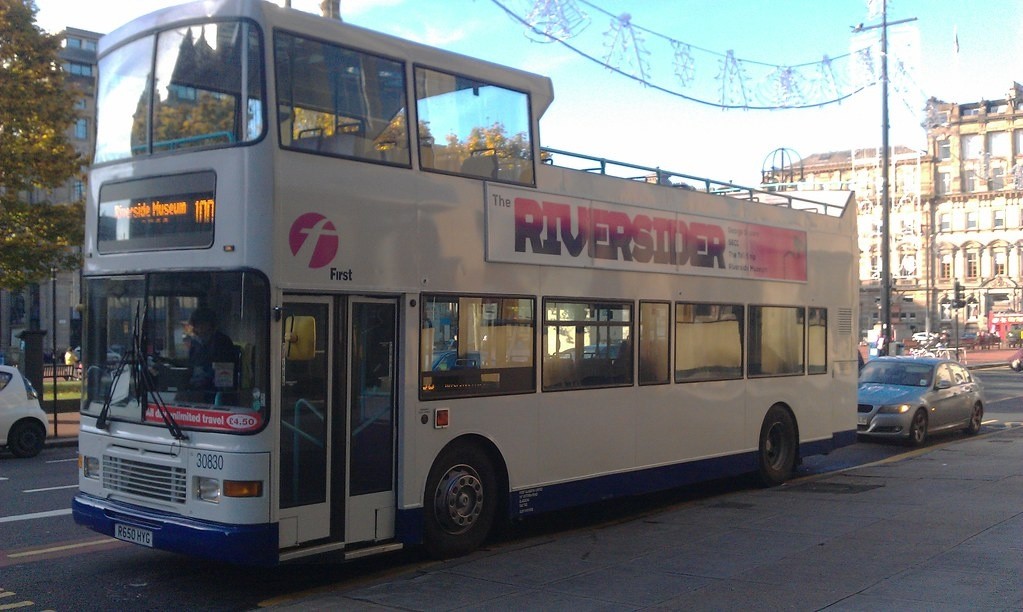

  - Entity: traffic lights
[950,280,966,308]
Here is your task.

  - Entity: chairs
[295,121,820,217]
[431,343,627,382]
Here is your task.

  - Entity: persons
[187,307,236,404]
[941,329,950,348]
[973,331,998,346]
[64,347,78,365]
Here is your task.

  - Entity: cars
[858,356,985,446]
[433,342,622,391]
[0,365,48,458]
[72,345,120,370]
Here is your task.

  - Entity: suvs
[962,332,998,345]
[912,332,942,346]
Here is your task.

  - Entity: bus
[71,0,860,574]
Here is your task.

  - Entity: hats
[67,346,73,351]
